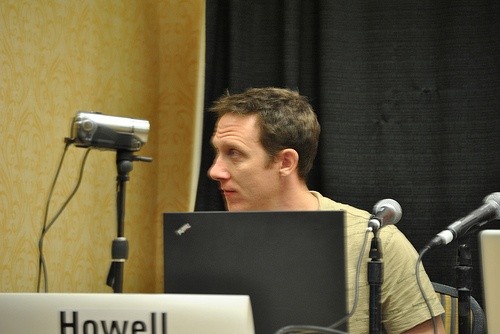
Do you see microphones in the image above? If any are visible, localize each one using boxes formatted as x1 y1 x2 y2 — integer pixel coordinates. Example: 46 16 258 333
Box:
366 199 403 232
426 192 500 246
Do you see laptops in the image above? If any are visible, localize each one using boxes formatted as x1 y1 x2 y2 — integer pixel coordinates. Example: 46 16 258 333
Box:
479 229 500 334
162 211 349 334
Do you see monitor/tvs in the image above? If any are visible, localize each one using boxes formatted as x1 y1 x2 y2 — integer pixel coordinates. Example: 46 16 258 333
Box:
0 293 255 333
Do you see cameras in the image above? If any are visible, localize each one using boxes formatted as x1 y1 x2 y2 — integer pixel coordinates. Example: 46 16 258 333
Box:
74 113 149 152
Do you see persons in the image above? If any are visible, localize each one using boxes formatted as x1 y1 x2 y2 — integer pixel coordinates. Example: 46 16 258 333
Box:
204 87 446 333
